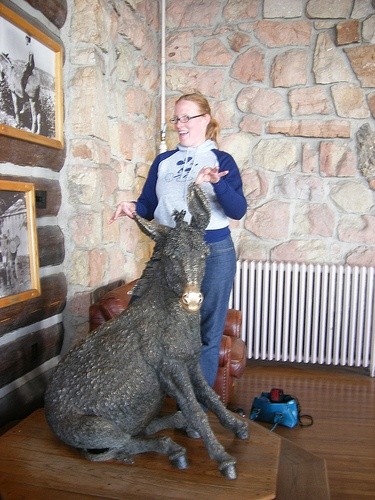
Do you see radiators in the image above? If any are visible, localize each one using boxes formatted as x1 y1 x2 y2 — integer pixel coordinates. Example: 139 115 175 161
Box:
226 258 375 378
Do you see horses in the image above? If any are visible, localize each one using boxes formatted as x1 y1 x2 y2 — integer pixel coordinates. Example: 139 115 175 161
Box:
44 181 249 481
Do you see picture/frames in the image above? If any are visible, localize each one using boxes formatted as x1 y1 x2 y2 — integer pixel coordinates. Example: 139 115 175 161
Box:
0 180 42 309
0 2 68 150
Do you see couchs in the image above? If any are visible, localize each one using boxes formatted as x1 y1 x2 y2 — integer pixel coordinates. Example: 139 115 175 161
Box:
88 278 248 410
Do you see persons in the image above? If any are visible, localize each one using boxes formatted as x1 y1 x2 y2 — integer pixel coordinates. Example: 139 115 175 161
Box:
108 94 247 439
21 36 35 103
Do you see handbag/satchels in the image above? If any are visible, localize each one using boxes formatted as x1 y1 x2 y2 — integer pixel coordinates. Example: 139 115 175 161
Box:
249 389 300 428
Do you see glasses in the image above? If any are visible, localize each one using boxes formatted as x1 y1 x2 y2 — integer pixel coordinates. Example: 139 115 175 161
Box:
169 113 207 124
